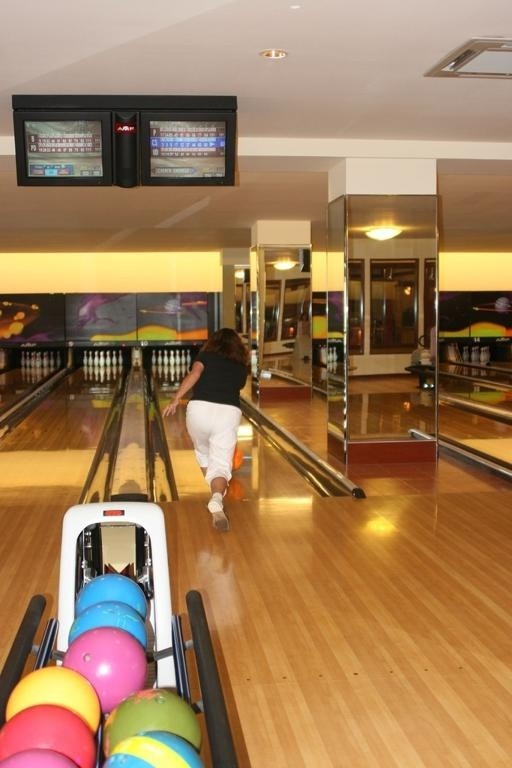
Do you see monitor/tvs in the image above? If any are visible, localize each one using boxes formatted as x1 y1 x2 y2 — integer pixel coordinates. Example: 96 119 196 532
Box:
13 111 113 186
140 111 236 187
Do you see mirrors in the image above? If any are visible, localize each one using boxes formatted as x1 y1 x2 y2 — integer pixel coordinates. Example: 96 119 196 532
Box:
233 265 252 369
345 193 438 444
262 244 312 388
423 257 436 350
369 258 419 355
326 196 346 439
349 258 365 355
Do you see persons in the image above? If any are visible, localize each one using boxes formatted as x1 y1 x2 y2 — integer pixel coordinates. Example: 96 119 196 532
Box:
160 328 250 533
300 312 310 362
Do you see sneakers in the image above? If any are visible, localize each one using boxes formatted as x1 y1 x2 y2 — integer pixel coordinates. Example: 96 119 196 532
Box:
208 498 229 531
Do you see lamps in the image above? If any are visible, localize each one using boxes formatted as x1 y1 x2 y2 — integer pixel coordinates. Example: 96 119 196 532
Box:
235 270 244 279
366 228 402 241
273 261 295 271
